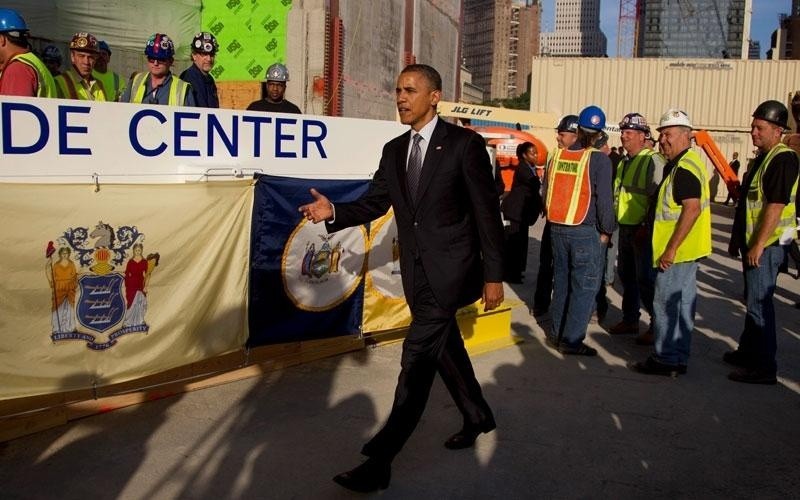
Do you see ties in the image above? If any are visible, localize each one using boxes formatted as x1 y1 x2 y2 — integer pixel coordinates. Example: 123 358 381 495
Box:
406 134 423 205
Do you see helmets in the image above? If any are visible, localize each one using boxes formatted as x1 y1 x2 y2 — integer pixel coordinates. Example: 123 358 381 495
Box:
752 100 792 130
190 31 219 53
69 32 100 52
0 7 30 32
41 46 63 63
98 41 112 54
264 63 289 82
655 108 693 131
619 113 650 133
554 115 578 132
144 33 175 58
577 106 606 131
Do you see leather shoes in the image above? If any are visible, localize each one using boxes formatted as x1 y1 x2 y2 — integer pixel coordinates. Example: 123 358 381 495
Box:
332 459 392 492
444 414 498 450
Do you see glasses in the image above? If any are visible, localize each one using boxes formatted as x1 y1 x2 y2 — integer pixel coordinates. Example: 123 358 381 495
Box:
148 56 171 64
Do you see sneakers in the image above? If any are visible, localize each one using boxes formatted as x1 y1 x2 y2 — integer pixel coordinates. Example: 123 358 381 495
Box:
634 326 657 345
728 367 777 386
722 350 752 363
559 342 596 357
607 313 640 335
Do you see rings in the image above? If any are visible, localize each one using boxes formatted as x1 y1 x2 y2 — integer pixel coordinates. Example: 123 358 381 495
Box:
497 304 500 306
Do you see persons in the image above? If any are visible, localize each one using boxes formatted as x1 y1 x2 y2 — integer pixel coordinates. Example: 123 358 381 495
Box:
0 8 57 98
246 63 301 114
298 64 511 493
41 45 63 76
180 32 219 108
122 243 148 327
91 40 126 101
53 31 107 102
46 247 78 334
119 33 196 107
499 101 799 384
